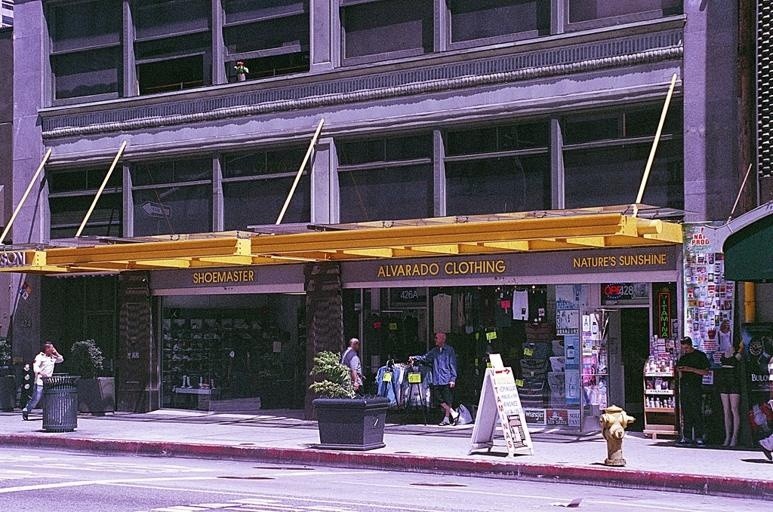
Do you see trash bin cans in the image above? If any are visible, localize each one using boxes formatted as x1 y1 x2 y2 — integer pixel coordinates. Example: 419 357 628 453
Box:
40 375 80 432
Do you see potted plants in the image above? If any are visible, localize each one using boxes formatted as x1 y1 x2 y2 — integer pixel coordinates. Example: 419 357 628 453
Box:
68 338 116 417
306 350 390 451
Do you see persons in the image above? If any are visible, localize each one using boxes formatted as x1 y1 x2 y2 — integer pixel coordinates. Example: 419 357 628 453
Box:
340 337 365 395
674 336 712 447
22 343 65 421
757 338 773 460
715 336 743 446
408 332 461 426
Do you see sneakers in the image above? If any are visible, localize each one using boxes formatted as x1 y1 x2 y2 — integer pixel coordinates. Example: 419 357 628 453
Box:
23 409 29 420
696 440 706 448
675 438 692 447
759 441 772 461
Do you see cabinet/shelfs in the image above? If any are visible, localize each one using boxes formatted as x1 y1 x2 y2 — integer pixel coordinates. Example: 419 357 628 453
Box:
642 358 677 431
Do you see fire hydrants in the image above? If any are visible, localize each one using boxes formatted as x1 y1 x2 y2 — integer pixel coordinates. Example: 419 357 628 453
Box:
598 403 637 467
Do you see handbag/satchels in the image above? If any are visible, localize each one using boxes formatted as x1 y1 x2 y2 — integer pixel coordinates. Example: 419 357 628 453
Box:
456 404 473 425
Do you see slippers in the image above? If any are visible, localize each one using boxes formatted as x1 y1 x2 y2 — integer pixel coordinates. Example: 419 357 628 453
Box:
453 413 460 426
439 421 450 426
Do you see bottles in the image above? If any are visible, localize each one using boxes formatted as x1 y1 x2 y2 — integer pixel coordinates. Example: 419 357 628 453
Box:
568 373 577 397
592 320 599 335
645 358 676 409
567 343 576 359
